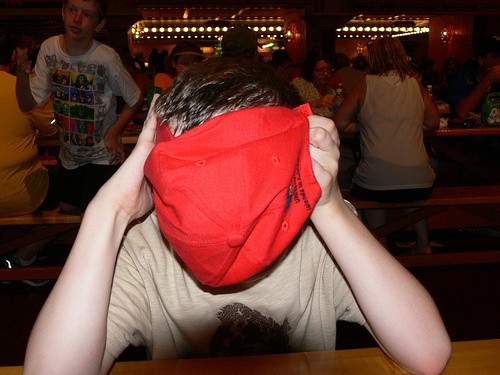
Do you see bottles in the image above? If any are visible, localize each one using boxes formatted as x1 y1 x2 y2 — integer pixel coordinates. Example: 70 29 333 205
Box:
481 83 500 127
332 81 345 115
426 84 435 104
127 51 150 73
136 98 148 125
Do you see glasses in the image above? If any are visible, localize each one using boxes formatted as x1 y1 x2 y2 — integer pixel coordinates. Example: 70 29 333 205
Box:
317 69 331 73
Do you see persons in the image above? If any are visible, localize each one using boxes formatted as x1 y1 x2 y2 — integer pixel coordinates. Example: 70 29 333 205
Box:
406 35 500 184
257 55 272 71
154 43 206 96
222 28 263 65
0 30 59 286
12 0 145 218
271 49 292 68
23 57 452 375
332 37 440 253
290 52 369 119
149 48 169 73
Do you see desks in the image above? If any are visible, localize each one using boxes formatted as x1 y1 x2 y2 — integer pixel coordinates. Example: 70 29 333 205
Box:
38 136 136 148
424 119 500 136
0 338 500 375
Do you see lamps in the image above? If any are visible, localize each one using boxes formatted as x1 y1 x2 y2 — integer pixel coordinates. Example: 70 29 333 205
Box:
439 24 451 45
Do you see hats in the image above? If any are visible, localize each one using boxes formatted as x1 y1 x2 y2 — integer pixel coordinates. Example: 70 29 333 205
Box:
143 103 322 289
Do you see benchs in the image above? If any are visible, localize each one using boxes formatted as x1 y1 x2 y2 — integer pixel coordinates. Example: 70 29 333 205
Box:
0 210 86 280
342 185 500 267
